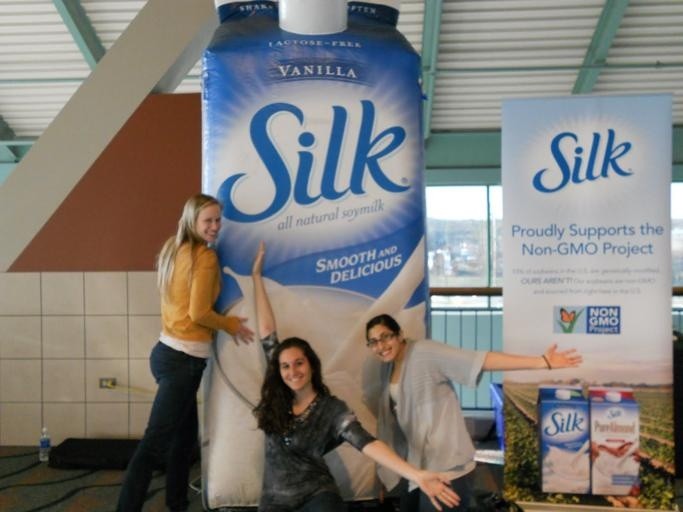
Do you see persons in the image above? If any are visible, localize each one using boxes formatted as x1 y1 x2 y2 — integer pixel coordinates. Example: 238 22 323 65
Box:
113 192 257 511
364 313 583 512
252 239 461 512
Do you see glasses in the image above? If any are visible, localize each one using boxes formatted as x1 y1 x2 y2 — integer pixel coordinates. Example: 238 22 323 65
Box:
366 332 396 347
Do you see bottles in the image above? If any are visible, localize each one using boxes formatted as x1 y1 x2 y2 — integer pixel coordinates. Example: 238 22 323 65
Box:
39 427 50 461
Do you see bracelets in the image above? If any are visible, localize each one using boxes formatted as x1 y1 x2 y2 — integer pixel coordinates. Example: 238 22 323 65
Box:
541 355 553 371
